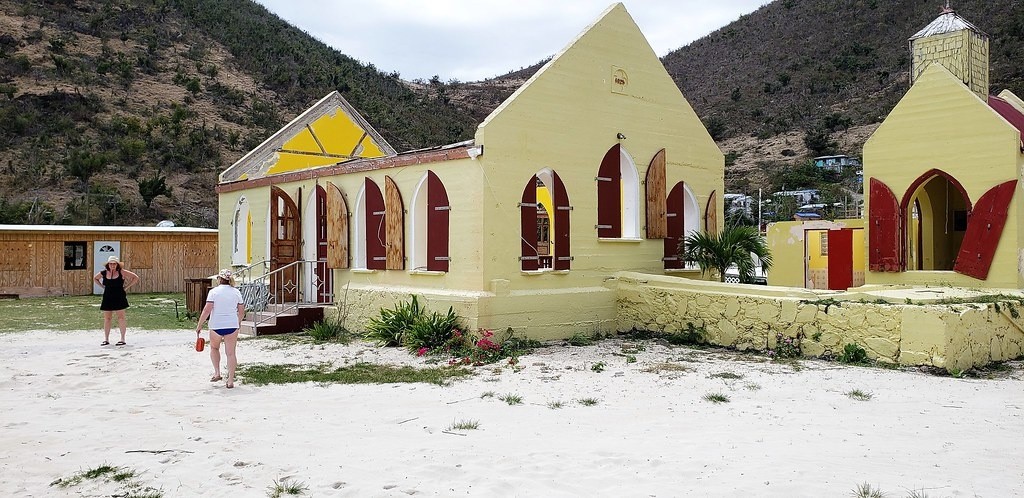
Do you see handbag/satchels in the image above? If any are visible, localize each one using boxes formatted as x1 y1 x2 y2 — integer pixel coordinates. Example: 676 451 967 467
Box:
196 338 205 351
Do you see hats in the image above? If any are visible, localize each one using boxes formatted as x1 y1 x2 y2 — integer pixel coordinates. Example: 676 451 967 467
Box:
219 269 231 281
101 256 124 269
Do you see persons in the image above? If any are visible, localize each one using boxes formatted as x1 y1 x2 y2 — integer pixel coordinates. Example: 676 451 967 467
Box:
195 268 245 389
92 255 140 346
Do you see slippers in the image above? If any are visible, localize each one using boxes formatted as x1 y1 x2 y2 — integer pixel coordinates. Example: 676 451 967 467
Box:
226 380 233 388
210 376 222 382
100 341 109 345
116 342 126 346
